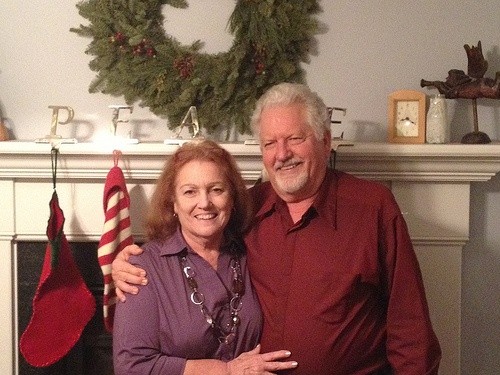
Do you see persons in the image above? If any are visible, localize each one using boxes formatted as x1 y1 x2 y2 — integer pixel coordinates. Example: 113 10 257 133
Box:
112 77 445 375
110 139 299 375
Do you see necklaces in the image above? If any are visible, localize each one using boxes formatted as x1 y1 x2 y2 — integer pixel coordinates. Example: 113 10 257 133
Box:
172 245 247 348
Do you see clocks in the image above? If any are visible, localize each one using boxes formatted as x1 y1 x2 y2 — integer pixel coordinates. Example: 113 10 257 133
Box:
389 89 426 143
